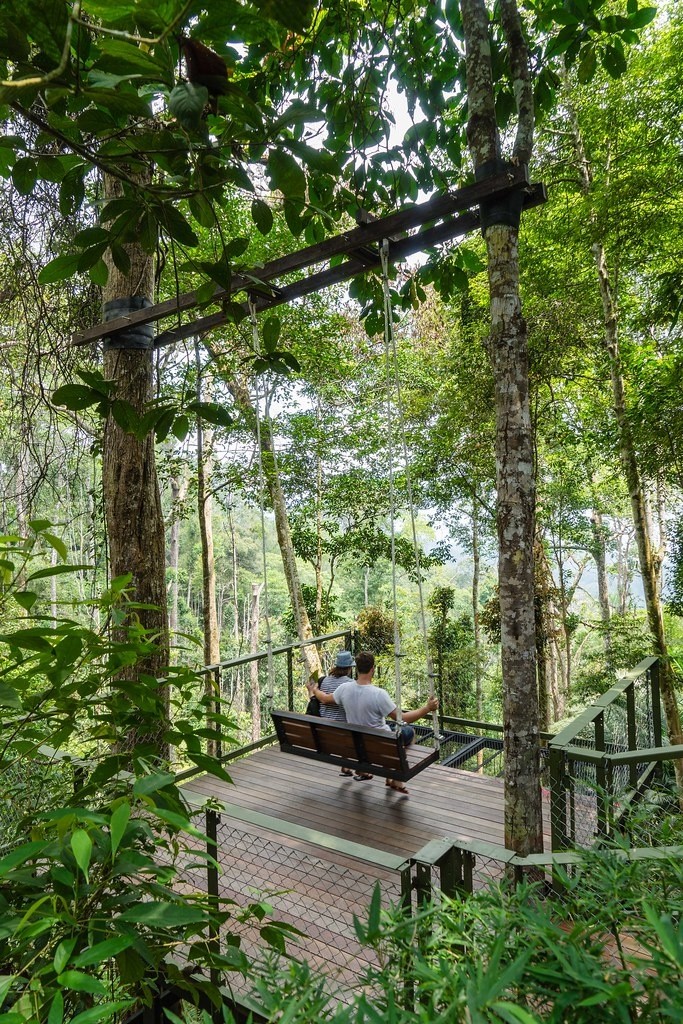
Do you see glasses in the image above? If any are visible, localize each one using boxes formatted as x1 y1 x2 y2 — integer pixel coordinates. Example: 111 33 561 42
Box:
373 665 376 668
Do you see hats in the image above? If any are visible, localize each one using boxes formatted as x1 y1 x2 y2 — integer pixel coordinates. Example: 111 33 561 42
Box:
333 651 357 667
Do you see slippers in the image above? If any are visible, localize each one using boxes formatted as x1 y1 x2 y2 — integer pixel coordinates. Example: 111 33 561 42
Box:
391 786 410 794
385 780 391 786
339 772 353 776
353 773 373 781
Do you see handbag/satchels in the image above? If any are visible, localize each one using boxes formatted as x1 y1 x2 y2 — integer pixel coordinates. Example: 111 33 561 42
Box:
306 676 327 717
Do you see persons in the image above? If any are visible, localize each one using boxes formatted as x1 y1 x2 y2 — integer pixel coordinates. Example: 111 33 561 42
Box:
313 652 439 794
318 651 372 781
306 677 325 717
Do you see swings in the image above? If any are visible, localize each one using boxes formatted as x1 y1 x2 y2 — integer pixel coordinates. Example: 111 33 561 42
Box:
245 237 444 782
